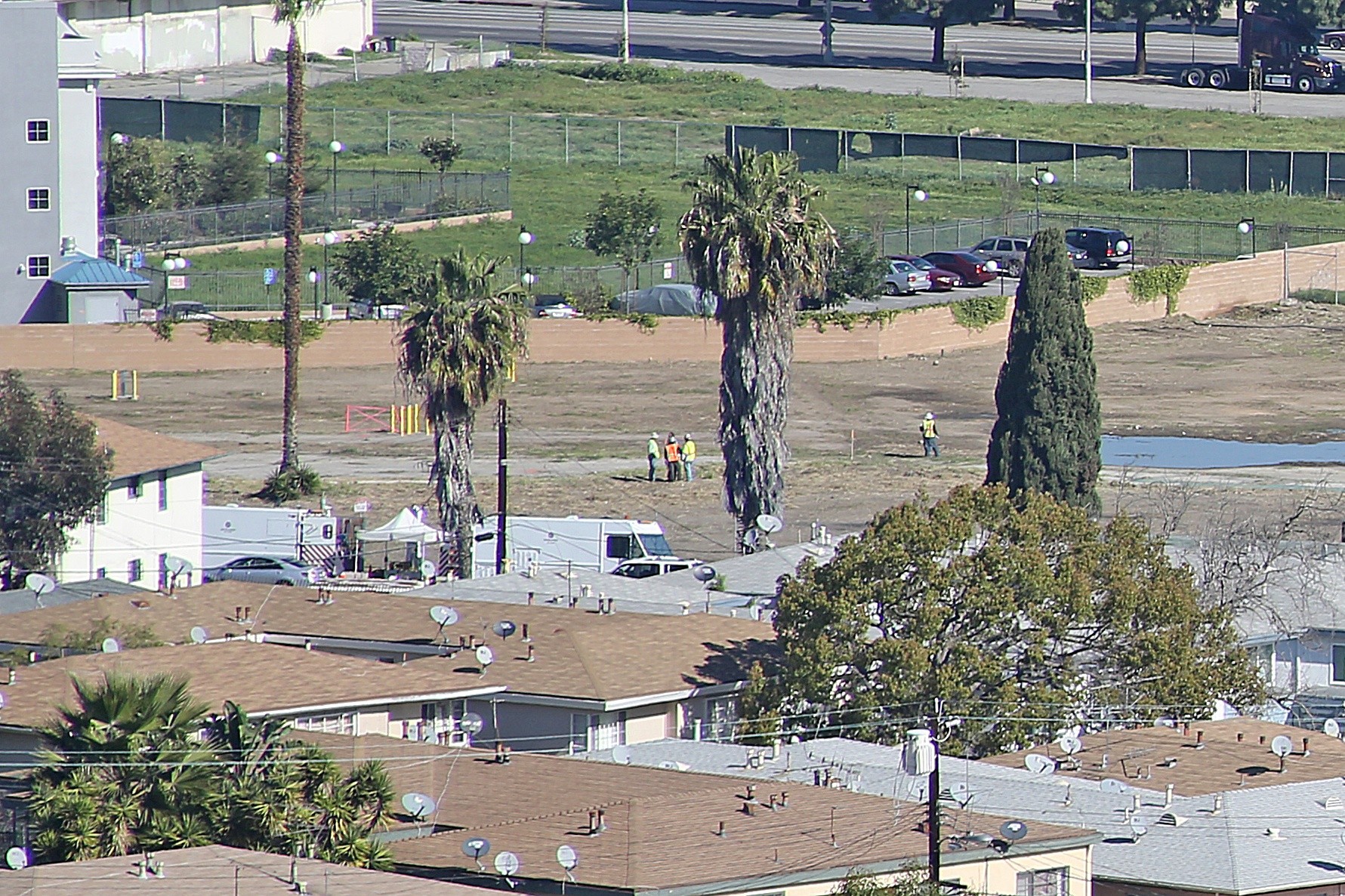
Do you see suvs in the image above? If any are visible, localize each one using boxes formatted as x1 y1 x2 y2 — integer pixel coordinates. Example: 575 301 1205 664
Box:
610 556 704 580
347 298 407 319
528 294 575 318
1065 226 1132 269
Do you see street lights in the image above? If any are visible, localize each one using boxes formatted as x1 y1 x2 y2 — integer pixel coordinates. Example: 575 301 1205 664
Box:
266 152 277 238
104 131 123 217
1030 166 1054 231
1236 217 1255 258
906 183 925 254
161 249 186 316
329 140 342 222
306 226 338 322
517 224 534 295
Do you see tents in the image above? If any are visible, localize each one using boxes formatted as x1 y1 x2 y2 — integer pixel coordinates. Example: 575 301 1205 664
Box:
354 508 457 581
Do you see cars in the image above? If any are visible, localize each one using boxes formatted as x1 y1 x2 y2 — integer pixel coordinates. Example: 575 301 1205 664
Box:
871 236 1088 296
157 302 207 322
203 554 327 587
1319 31 1345 49
610 284 718 317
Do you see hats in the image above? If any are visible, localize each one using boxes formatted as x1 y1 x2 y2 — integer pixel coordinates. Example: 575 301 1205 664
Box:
925 412 934 420
650 432 659 438
684 434 692 440
669 436 677 442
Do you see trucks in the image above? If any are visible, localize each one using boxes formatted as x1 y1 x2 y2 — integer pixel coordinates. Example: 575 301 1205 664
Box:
472 515 671 580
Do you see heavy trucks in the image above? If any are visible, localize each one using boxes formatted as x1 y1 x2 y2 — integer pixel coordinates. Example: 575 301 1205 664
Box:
1180 13 1342 92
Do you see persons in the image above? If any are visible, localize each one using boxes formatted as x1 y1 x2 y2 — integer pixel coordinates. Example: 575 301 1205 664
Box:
920 412 940 458
664 432 695 482
647 432 660 482
